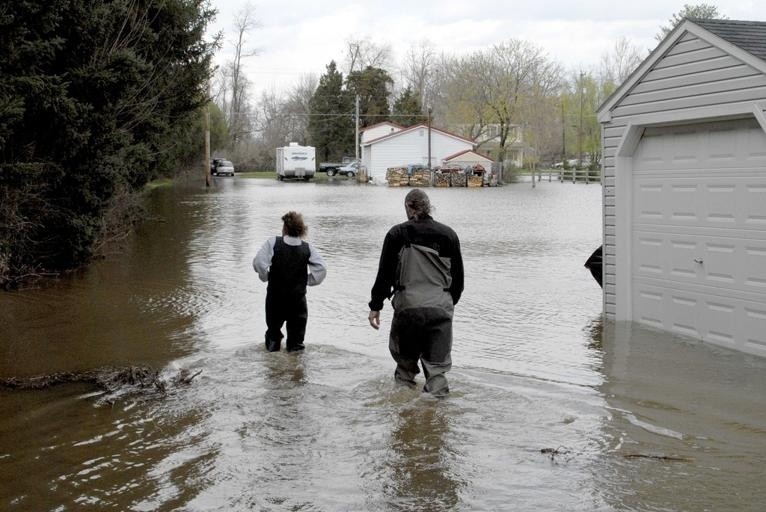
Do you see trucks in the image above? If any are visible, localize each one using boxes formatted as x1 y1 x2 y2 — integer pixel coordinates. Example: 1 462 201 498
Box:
274 141 316 181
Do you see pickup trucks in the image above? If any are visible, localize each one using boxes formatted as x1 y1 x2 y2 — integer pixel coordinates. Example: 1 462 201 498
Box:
319 156 354 176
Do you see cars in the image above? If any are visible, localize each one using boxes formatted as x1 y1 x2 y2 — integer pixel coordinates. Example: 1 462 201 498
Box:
338 161 358 177
211 157 235 177
554 159 578 169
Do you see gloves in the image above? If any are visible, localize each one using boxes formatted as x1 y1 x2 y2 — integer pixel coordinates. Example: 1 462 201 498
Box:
368 311 380 329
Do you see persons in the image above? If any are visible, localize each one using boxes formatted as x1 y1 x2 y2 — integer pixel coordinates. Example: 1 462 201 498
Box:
251 210 328 354
366 188 465 399
